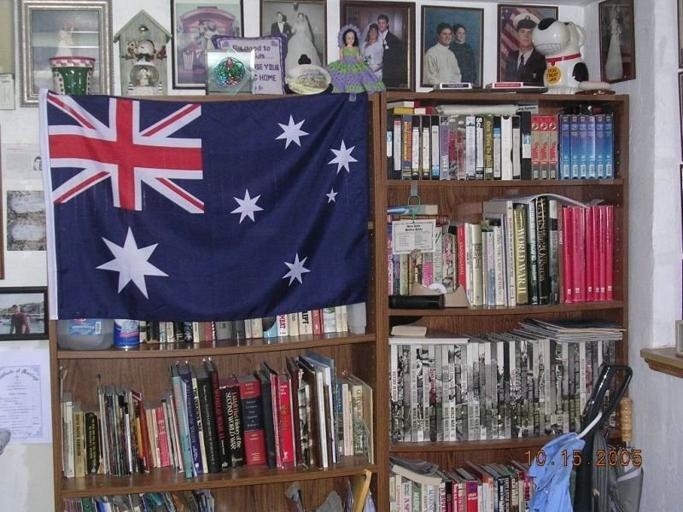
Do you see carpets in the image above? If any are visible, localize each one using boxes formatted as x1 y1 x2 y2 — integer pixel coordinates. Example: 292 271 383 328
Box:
517 55 525 81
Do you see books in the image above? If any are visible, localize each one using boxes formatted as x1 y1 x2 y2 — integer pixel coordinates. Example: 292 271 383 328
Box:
387 194 619 309
387 101 618 181
57 305 367 349
61 353 375 510
387 318 625 510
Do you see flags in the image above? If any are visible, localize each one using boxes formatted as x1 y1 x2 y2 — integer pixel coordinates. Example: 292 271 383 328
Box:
38 89 370 321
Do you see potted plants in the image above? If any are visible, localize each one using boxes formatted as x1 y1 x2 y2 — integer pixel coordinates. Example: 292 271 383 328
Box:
0 286 48 340
171 0 244 89
260 1 327 69
421 5 484 88
340 0 416 92
211 35 286 96
598 1 636 83
13 1 113 108
204 49 255 94
497 3 558 86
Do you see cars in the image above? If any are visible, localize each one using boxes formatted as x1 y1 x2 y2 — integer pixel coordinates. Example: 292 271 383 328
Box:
48 56 96 96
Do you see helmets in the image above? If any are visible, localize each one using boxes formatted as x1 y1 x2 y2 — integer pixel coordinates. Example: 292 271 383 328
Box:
286 64 331 95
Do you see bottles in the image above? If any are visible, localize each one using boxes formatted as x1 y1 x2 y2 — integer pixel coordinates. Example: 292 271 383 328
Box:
513 13 540 32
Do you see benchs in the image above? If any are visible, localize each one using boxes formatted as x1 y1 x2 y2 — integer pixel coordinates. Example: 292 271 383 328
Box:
38 89 370 321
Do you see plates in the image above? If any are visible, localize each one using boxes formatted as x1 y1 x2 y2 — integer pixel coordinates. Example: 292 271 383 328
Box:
379 92 629 511
41 93 385 512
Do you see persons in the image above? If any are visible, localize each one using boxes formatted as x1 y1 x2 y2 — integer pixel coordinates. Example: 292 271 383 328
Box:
448 24 477 84
10 304 30 334
604 5 624 82
504 12 546 87
423 23 462 86
270 12 292 81
360 14 403 88
285 13 321 85
